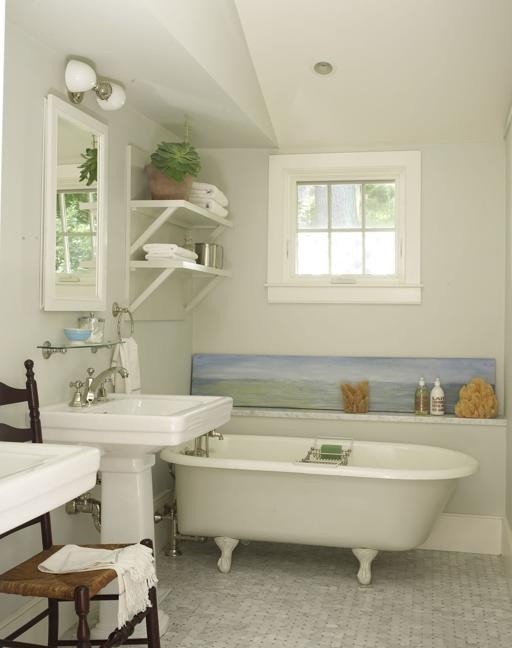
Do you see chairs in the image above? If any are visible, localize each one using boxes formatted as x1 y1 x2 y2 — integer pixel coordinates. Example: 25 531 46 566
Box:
0 359 161 647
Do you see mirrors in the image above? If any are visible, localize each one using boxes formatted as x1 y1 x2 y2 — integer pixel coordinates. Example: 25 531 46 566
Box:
40 94 109 314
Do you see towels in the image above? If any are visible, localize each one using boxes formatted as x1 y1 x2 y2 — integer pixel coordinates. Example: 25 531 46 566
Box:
36 544 159 631
143 244 199 264
189 182 229 217
111 338 143 395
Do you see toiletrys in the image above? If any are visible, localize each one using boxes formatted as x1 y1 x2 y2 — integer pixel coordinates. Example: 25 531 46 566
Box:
430 375 447 416
413 375 431 415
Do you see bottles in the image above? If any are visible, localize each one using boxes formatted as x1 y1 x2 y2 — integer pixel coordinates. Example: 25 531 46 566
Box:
88 312 97 343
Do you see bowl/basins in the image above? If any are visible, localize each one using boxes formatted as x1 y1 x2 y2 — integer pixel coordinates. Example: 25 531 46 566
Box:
194 243 224 269
64 328 91 346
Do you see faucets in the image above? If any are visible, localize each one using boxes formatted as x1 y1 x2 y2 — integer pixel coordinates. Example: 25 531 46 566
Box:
194 429 223 455
80 364 130 406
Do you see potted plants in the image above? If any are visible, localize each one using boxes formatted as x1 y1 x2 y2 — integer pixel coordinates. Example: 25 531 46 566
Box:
146 140 204 200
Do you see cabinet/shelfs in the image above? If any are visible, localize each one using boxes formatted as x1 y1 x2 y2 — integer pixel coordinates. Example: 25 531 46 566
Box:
125 145 232 322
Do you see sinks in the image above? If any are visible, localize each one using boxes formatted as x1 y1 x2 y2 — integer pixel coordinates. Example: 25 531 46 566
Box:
0 440 101 537
25 391 234 455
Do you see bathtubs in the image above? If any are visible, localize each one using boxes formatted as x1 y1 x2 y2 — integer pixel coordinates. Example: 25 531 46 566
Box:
159 434 479 551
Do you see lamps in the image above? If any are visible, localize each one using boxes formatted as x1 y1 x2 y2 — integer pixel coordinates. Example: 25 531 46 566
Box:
64 58 126 112
